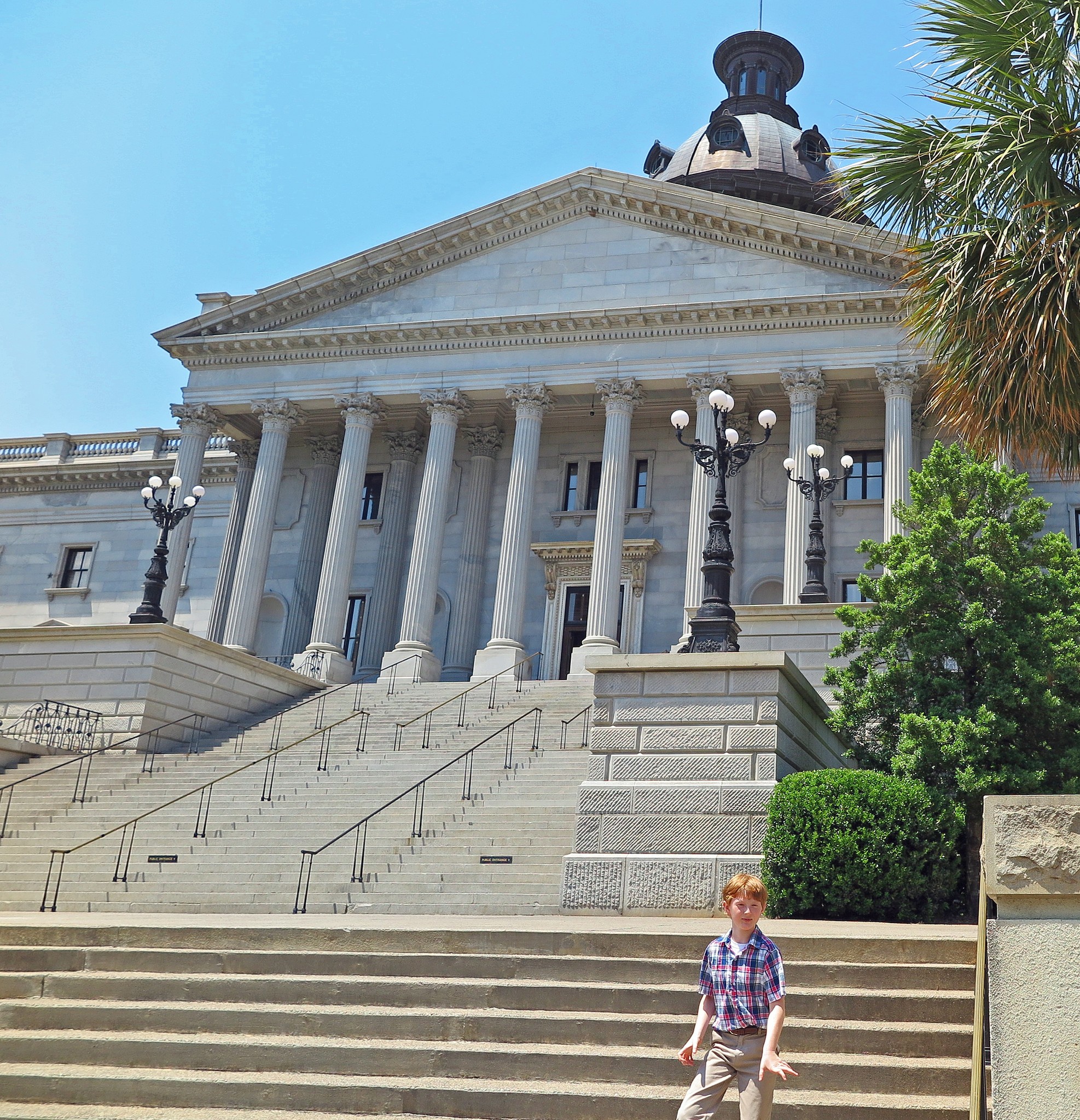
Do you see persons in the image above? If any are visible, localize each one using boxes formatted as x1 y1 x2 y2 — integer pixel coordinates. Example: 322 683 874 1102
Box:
674 873 799 1120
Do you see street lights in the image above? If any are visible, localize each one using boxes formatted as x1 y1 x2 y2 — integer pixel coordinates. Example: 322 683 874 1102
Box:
127 474 203 625
669 388 778 652
782 443 855 602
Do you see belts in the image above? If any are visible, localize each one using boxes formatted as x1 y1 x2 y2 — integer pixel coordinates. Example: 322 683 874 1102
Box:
729 1027 759 1036
